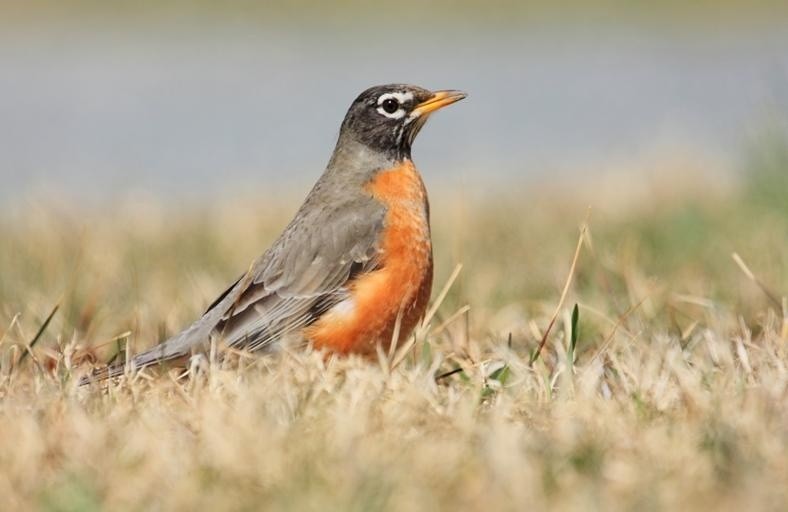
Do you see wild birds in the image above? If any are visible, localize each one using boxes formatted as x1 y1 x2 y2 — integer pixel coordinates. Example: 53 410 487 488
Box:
77 82 470 389
